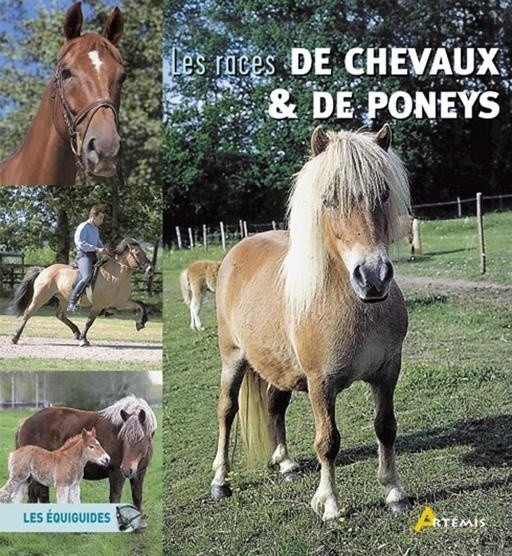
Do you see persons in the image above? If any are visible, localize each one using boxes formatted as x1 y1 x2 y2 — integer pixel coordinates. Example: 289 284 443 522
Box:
66 203 112 313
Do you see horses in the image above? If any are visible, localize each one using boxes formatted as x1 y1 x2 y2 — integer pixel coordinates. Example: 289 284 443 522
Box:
12 392 157 513
207 123 416 525
0 1 129 185
0 427 111 505
179 258 221 334
9 236 156 349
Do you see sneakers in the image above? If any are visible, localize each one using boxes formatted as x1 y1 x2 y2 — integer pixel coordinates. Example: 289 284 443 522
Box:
66 304 78 312
